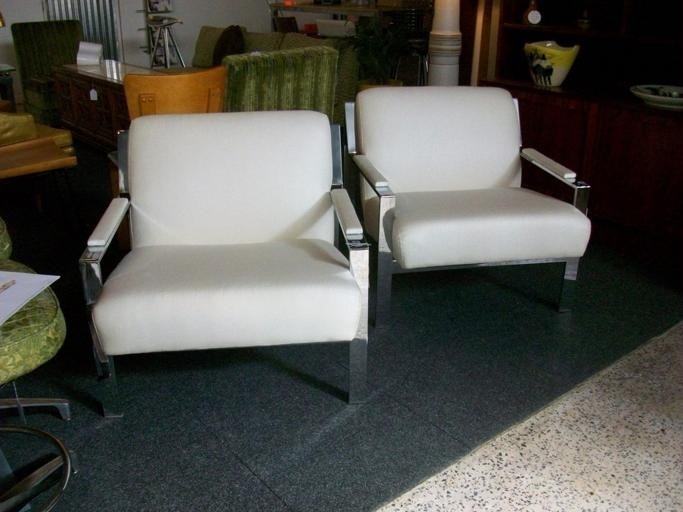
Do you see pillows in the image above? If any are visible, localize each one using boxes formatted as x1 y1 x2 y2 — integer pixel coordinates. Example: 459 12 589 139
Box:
211 25 245 67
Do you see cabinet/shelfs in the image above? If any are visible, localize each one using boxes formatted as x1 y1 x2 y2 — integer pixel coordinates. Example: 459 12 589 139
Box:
494 0 682 96
477 67 682 294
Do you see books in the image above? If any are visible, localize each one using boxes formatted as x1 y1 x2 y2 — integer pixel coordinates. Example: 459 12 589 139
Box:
0 271 62 331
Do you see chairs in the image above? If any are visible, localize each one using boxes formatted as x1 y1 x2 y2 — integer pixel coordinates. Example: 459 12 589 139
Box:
0 219 71 427
10 19 84 129
345 82 591 330
79 110 371 406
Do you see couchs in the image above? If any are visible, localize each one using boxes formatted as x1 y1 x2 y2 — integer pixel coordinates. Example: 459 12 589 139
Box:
153 25 359 147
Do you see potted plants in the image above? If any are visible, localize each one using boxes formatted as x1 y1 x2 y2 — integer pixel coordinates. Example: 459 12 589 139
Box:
349 7 429 92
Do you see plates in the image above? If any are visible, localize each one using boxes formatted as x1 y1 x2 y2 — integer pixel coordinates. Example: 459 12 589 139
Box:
631 85 683 104
